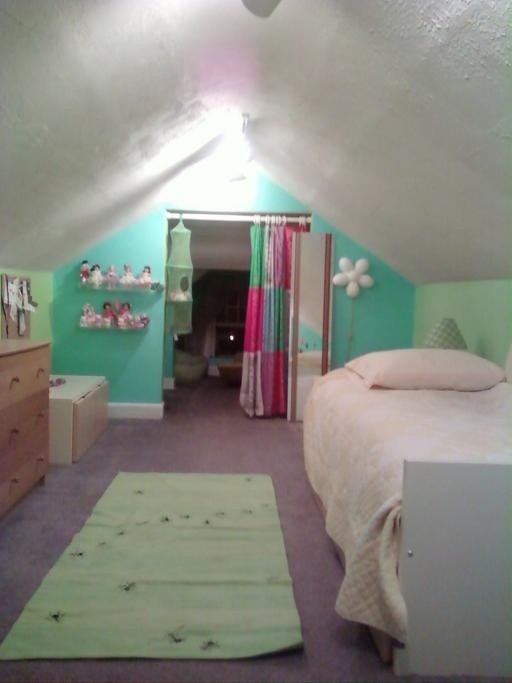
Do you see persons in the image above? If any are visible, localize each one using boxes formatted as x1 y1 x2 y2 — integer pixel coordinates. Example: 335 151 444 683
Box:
78 258 152 330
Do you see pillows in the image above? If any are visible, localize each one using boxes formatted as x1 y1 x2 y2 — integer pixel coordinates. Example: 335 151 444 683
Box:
343 348 507 391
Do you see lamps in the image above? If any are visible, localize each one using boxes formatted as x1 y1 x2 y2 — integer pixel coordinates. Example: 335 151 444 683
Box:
424 317 469 351
332 257 375 365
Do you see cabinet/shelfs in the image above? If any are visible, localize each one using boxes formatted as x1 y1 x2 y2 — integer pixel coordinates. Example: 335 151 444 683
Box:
49 375 109 465
0 338 51 521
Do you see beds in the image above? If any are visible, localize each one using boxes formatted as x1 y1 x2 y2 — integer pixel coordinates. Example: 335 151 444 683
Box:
303 367 512 683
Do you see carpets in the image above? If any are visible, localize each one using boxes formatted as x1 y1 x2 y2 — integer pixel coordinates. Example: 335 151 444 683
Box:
0 471 305 661
